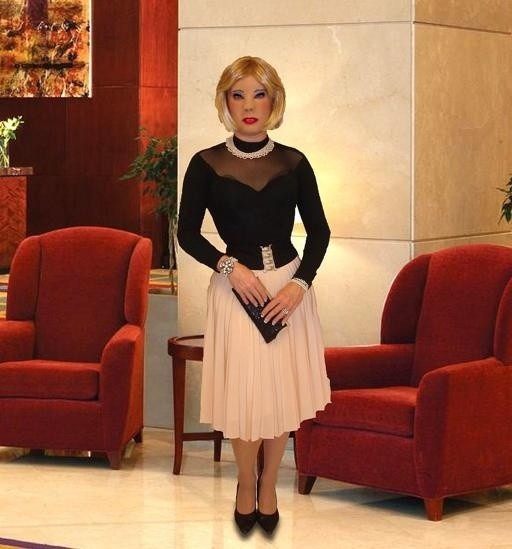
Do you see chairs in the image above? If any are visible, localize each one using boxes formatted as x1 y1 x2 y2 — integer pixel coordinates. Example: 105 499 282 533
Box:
1 226 150 471
293 242 510 520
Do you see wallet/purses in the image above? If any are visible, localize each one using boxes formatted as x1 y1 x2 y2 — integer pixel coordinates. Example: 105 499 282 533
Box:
231 278 287 343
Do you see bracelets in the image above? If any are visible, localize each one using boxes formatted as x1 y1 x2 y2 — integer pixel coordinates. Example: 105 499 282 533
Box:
292 278 310 294
216 256 239 278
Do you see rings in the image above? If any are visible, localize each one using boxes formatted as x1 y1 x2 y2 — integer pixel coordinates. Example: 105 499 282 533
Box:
282 309 289 315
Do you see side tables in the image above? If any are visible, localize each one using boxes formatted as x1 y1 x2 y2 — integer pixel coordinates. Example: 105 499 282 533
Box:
169 334 228 477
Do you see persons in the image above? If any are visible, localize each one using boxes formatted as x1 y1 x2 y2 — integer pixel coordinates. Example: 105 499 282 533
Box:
177 54 331 536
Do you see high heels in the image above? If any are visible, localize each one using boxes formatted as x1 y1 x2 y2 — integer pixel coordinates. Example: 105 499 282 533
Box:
234 470 279 535
